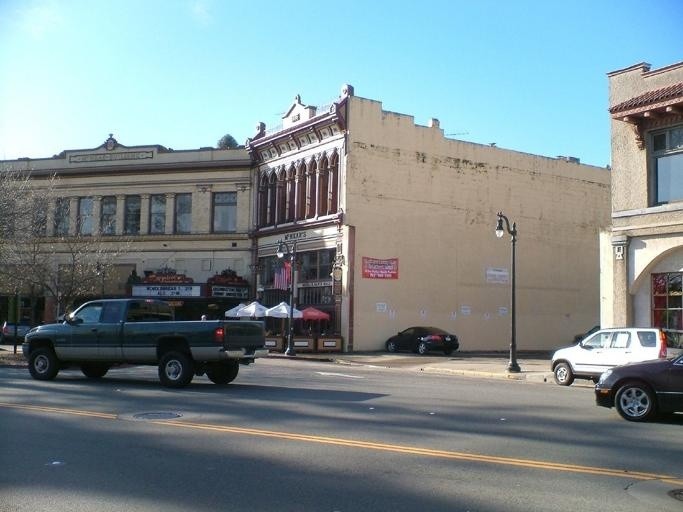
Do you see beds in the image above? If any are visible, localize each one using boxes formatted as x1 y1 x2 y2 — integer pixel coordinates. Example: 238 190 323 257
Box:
225 301 330 334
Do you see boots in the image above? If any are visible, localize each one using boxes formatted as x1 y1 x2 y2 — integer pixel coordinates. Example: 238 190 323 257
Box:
549 328 682 387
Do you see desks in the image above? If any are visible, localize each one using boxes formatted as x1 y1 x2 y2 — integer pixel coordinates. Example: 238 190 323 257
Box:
274 239 300 359
494 211 522 374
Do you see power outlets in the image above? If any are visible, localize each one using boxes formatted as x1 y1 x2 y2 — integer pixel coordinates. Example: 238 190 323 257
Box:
22 296 270 391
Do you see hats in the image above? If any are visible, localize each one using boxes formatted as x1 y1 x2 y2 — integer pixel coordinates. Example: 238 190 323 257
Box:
384 326 460 356
2 322 30 339
595 354 682 421
